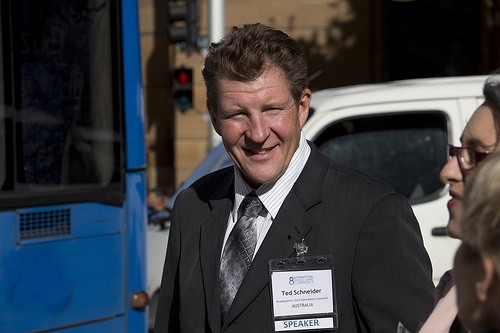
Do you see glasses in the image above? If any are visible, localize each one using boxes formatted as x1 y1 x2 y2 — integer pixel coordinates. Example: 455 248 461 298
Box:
445 144 499 171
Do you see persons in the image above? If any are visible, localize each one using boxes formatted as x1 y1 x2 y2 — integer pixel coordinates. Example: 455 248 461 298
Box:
152 24 438 333
418 73 500 333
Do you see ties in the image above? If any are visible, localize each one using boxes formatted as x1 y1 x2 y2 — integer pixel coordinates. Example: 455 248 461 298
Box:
218 189 263 325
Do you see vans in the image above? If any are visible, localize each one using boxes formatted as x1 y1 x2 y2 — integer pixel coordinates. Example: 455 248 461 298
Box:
147 76 500 331
